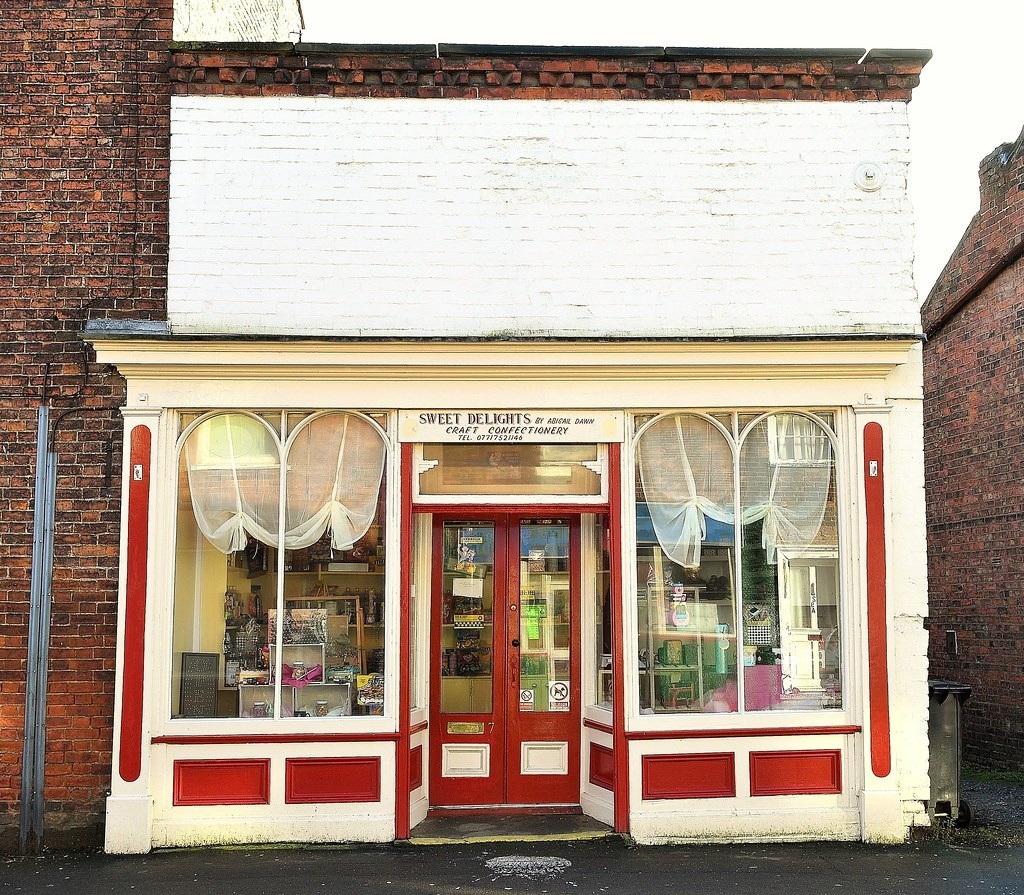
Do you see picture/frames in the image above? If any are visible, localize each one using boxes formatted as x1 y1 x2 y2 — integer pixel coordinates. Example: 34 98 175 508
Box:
180 652 220 717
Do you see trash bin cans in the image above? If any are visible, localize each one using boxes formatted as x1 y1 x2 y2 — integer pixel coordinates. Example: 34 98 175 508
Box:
928 680 975 827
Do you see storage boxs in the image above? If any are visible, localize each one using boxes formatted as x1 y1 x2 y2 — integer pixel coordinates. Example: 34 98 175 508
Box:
357 675 384 706
269 643 326 684
328 563 370 572
238 684 295 718
295 684 351 716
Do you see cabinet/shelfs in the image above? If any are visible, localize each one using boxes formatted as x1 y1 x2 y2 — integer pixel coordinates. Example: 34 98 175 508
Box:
172 511 736 717
